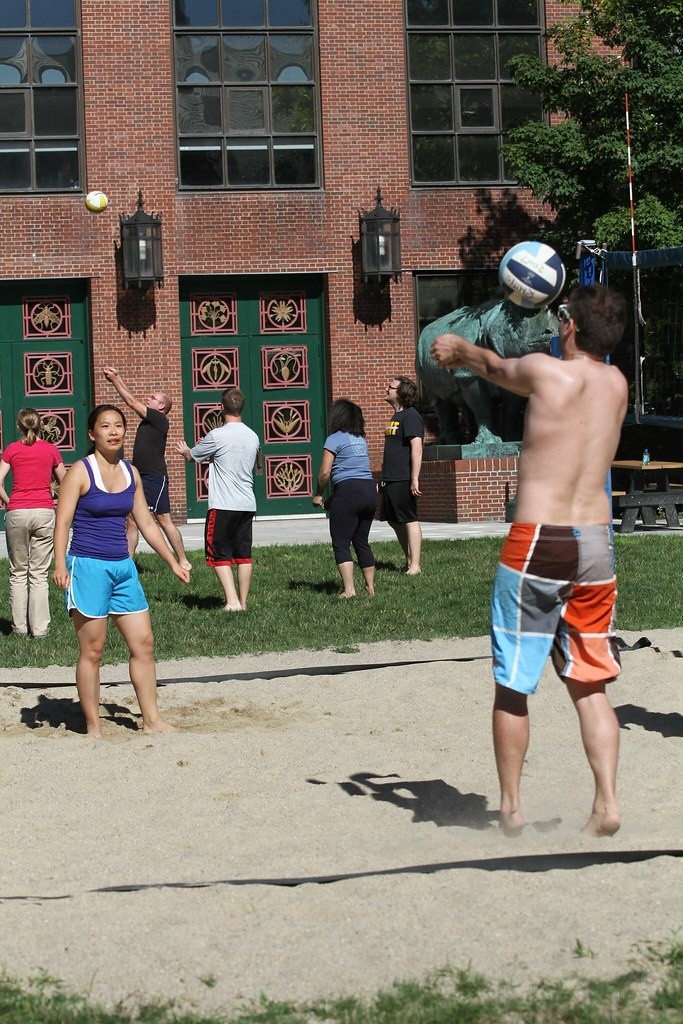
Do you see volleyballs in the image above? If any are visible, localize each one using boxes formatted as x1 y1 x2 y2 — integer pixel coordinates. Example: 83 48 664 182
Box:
84 191 108 213
496 240 567 310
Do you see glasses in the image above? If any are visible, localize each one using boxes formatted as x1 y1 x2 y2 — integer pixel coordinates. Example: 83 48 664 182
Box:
388 385 397 390
557 304 579 331
150 395 164 404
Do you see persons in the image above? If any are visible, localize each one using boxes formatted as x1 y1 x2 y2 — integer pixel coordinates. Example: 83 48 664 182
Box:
430 284 629 837
311 400 377 599
50 404 190 737
175 388 259 611
380 375 425 574
103 367 192 571
0 408 66 636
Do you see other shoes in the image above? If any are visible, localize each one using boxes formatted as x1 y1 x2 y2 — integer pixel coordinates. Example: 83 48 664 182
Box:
35 635 45 639
13 633 27 637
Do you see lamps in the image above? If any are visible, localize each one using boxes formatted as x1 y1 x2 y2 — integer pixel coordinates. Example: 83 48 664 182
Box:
358 184 403 294
114 190 164 300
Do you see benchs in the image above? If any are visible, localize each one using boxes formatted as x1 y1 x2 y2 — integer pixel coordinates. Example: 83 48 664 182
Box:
611 483 683 534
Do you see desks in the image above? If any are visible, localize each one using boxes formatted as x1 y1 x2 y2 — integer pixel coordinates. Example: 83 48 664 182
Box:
611 460 683 524
